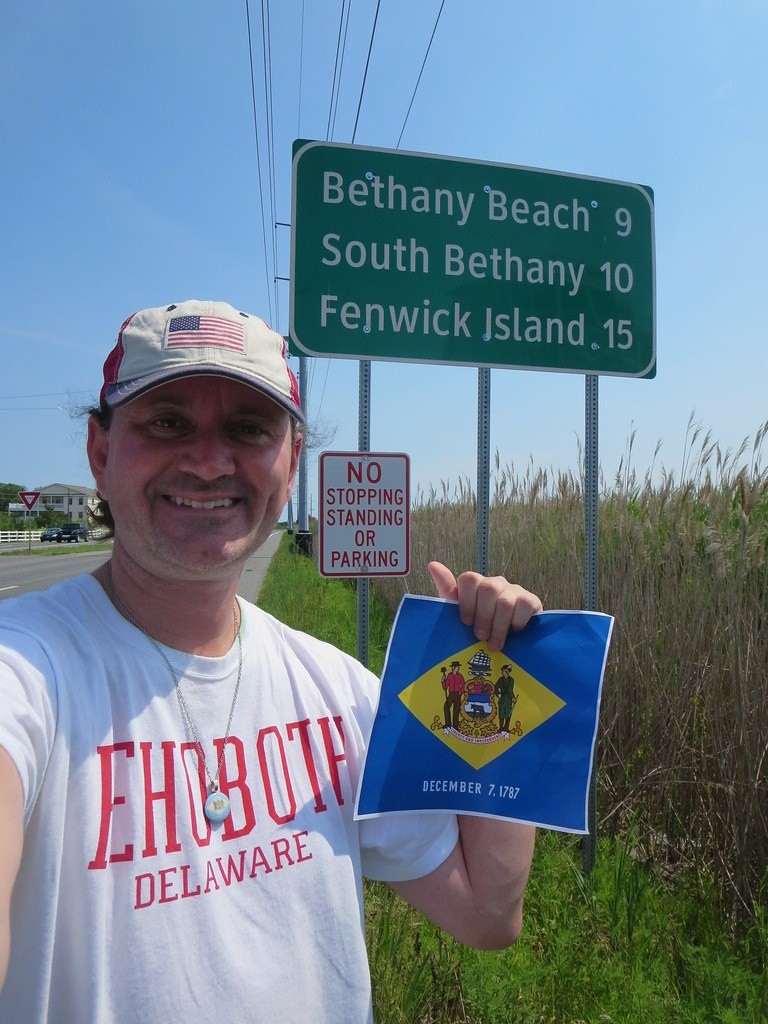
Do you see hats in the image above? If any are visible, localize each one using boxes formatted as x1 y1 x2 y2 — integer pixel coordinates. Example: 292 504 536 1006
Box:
100 300 305 427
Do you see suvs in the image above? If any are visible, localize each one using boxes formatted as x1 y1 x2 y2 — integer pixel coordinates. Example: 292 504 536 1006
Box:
57 522 89 542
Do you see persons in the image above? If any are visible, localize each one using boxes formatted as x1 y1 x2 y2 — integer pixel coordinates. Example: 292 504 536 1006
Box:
0 299 544 1024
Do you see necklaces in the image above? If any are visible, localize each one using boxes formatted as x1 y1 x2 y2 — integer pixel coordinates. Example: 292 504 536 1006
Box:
106 559 243 823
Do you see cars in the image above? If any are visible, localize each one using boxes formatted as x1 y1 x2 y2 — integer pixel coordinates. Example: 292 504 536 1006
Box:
40 528 61 542
